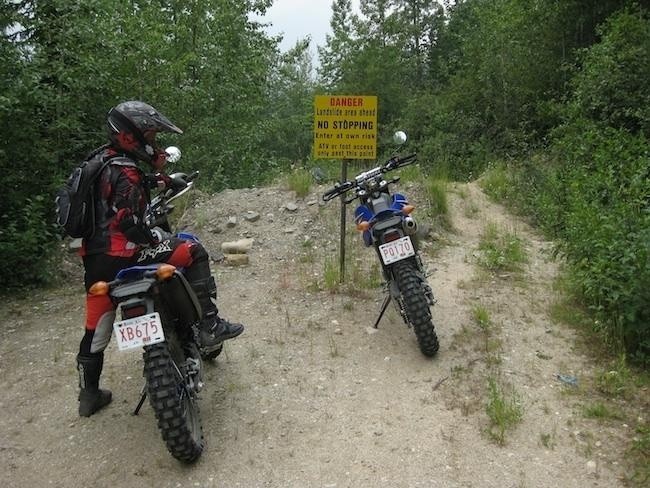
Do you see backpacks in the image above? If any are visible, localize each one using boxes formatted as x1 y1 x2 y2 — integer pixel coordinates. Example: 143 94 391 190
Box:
52 142 137 239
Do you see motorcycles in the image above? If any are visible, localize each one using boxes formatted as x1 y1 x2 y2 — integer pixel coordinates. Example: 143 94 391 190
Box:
68 144 207 467
321 130 441 359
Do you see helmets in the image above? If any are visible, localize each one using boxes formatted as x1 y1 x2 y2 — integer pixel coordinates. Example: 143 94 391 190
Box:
108 100 183 170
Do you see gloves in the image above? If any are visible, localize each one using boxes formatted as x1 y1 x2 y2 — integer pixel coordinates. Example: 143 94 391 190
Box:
151 225 174 244
168 172 188 189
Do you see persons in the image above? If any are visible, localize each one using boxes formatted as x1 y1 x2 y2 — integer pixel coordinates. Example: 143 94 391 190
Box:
72 100 244 418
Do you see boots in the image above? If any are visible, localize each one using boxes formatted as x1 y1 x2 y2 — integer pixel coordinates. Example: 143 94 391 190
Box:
76 352 113 417
192 276 244 346
